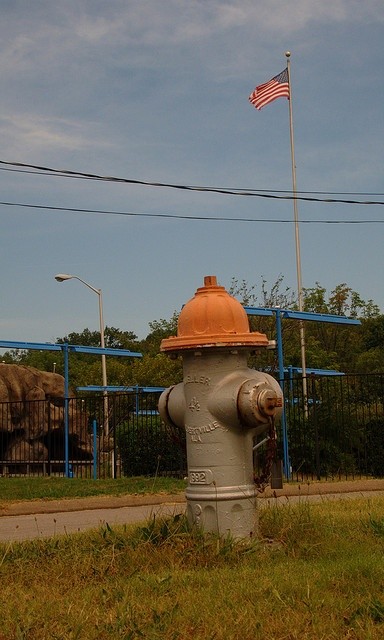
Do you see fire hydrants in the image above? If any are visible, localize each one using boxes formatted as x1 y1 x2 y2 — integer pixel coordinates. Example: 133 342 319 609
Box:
159 276 284 546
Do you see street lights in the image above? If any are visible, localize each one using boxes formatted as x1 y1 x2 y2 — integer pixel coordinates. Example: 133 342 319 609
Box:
55 274 110 480
